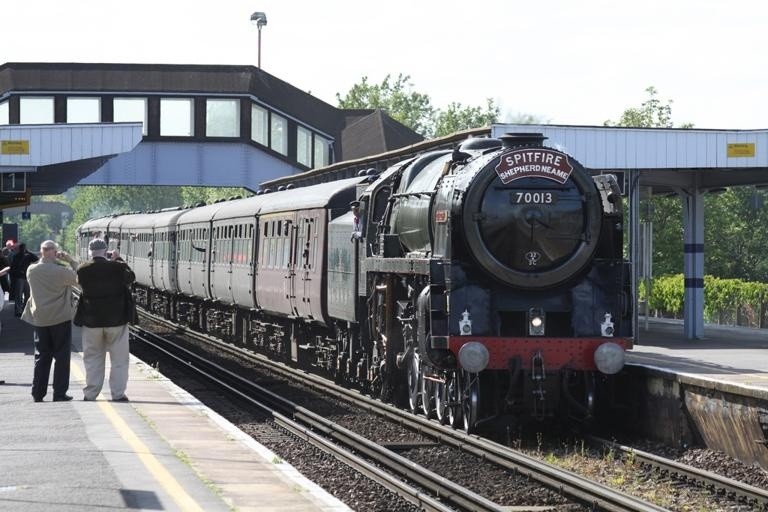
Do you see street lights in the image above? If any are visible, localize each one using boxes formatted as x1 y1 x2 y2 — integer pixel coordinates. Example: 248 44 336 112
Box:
249 7 271 69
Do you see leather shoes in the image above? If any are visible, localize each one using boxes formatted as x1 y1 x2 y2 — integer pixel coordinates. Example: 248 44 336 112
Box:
53 395 73 401
113 395 128 401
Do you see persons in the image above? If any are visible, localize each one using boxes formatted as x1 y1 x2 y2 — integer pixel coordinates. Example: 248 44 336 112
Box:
76 239 139 402
0 239 39 317
21 241 80 402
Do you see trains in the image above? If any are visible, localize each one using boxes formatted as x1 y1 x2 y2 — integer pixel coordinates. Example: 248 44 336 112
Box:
73 125 635 448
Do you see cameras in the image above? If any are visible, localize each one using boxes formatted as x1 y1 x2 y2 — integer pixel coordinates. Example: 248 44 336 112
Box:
56 253 65 258
107 252 112 258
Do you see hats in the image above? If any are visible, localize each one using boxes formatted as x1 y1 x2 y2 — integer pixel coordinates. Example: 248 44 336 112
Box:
89 239 106 250
41 240 58 250
